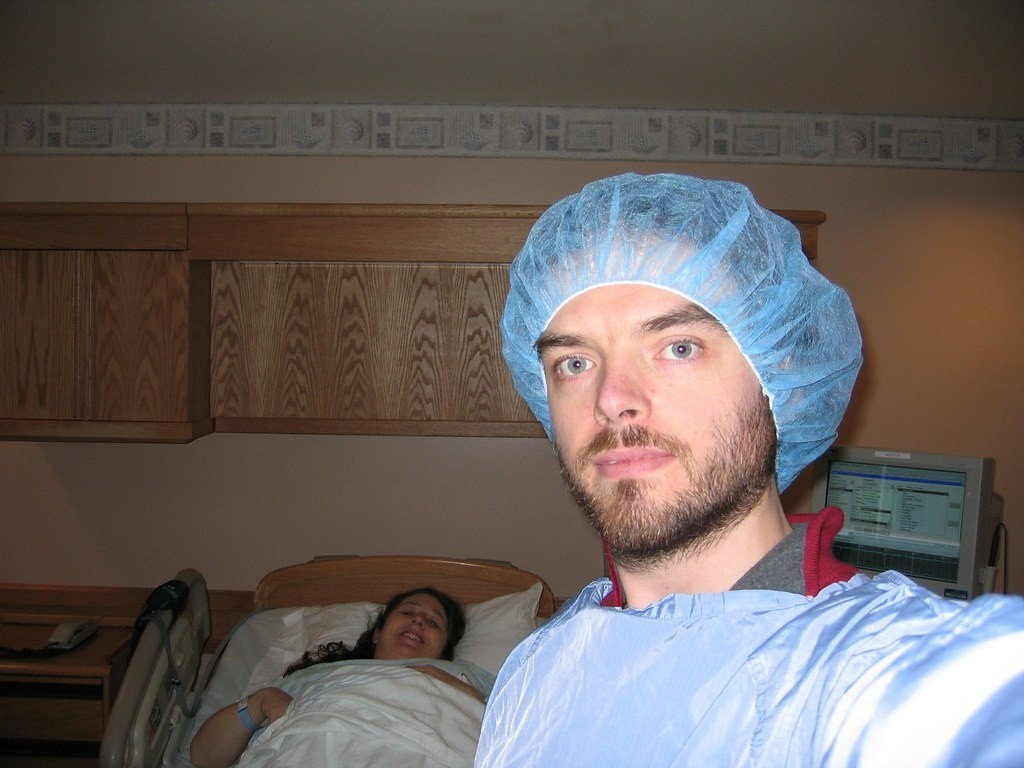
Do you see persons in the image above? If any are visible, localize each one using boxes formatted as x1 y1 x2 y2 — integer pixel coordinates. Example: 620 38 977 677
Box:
474 174 1024 768
190 585 496 768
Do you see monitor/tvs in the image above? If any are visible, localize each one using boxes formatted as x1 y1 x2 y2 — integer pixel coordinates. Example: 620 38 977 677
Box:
811 445 1004 602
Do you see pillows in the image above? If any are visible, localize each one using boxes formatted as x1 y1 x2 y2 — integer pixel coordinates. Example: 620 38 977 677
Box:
302 581 543 677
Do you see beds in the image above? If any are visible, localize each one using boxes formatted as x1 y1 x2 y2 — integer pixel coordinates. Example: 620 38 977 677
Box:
97 554 556 768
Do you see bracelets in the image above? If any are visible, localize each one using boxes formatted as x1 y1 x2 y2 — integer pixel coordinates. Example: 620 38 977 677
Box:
237 697 268 731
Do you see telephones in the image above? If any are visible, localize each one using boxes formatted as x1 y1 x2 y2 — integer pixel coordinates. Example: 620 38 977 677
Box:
47 619 99 650
